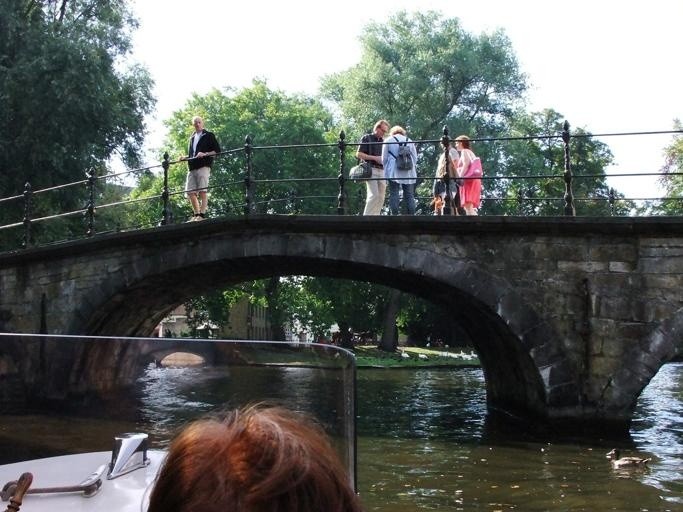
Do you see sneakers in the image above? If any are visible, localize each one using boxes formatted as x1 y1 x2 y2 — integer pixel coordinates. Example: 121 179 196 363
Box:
185 216 206 223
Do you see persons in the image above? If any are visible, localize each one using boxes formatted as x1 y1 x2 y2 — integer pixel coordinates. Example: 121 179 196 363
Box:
354 117 390 216
380 125 417 214
453 134 482 216
176 114 222 222
136 396 367 512
431 133 458 215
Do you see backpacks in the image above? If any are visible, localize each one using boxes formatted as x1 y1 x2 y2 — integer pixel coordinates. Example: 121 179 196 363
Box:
388 136 414 171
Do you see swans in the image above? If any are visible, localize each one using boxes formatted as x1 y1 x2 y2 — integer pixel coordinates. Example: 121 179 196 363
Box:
419 351 429 360
401 346 410 359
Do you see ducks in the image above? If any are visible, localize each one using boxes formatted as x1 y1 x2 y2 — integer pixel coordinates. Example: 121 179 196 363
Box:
606 448 652 471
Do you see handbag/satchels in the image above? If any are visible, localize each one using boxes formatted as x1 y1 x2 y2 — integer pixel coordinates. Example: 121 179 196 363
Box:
456 155 483 178
349 161 372 183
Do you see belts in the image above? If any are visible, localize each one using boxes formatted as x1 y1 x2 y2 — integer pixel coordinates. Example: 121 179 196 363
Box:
371 164 383 169
